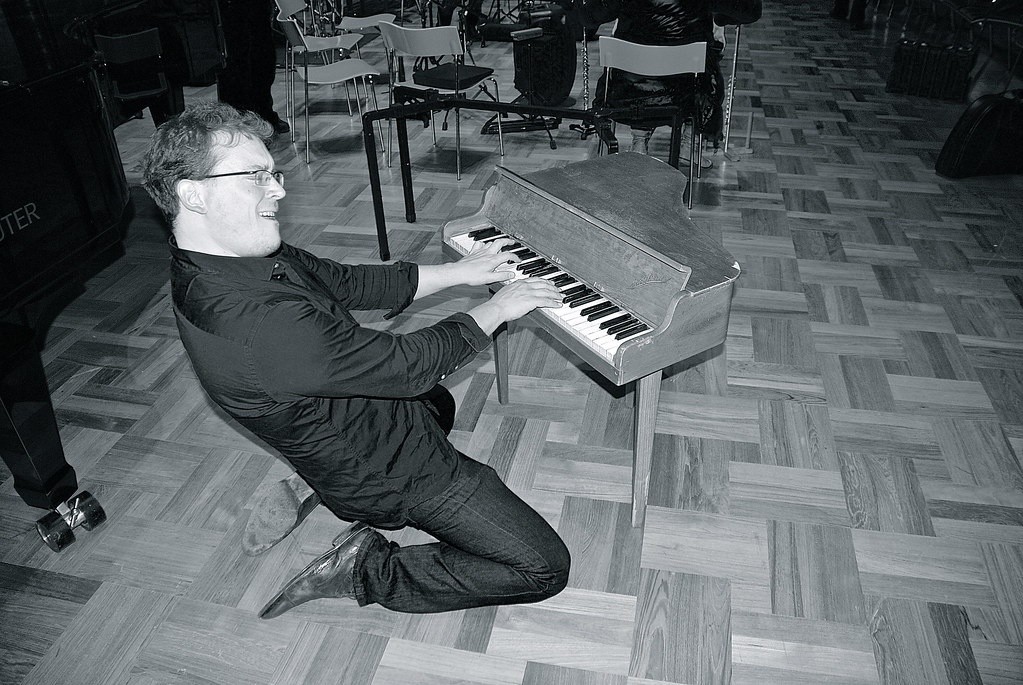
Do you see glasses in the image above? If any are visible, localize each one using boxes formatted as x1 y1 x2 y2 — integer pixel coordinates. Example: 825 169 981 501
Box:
207 169 285 188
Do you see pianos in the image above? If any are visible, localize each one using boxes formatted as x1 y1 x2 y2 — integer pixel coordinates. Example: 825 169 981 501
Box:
1 0 291 553
442 149 741 530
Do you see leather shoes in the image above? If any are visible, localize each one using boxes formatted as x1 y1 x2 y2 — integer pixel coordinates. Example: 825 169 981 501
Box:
257 519 372 620
243 470 322 553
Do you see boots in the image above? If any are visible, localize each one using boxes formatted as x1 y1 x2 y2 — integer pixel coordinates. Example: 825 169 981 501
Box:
679 121 714 170
629 128 652 155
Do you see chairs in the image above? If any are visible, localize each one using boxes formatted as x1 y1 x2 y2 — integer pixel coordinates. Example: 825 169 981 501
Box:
275 0 707 208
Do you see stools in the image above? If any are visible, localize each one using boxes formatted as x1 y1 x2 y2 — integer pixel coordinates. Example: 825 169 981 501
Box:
481 24 527 48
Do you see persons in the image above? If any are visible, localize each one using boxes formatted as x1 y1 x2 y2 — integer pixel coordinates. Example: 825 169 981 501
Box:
436 0 484 41
215 0 290 134
135 104 572 619
565 0 762 169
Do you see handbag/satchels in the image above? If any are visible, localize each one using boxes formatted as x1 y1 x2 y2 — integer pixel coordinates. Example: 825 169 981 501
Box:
936 88 1023 179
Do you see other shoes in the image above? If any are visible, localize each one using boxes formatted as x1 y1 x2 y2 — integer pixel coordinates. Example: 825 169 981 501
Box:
830 9 846 19
277 117 290 133
850 21 872 31
